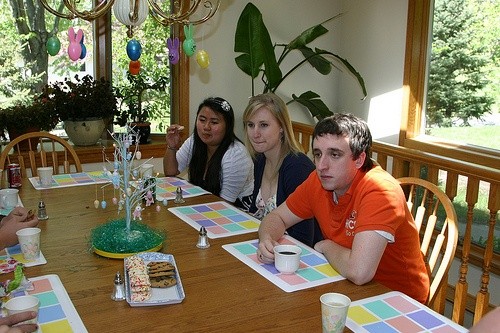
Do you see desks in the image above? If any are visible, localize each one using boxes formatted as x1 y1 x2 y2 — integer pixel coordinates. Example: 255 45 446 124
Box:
0 172 394 333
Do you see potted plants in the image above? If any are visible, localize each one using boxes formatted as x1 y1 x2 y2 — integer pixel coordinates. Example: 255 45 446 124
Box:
111 71 169 144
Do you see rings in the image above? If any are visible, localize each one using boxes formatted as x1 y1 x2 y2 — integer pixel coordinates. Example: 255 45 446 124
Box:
260 255 263 259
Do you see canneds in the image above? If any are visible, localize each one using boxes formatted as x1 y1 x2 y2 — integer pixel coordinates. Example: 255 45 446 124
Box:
7 163 22 189
141 176 157 203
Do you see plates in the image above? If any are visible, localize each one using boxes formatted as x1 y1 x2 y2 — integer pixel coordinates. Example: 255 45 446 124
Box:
123 250 186 307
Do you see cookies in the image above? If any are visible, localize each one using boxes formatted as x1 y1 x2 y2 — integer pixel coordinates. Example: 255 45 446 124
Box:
126 256 152 303
147 261 177 288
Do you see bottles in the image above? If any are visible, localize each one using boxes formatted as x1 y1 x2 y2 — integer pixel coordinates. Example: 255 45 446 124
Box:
111 271 125 302
196 226 210 249
37 200 48 221
174 187 185 203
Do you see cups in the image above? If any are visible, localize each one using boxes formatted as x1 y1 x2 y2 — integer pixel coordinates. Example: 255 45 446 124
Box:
36 167 53 187
272 244 302 275
15 227 42 261
4 295 40 333
320 292 351 333
0 188 19 209
141 164 153 180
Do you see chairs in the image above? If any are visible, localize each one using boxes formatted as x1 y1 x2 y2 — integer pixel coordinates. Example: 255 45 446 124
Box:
396 177 459 308
0 132 83 179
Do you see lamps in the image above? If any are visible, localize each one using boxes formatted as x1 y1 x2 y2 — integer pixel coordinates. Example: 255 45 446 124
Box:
40 0 221 39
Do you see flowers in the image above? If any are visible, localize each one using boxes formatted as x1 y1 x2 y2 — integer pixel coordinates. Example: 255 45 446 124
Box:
0 74 121 141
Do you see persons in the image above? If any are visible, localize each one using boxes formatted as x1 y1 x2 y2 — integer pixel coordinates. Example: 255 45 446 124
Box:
0 207 39 251
256 113 429 304
242 93 316 249
163 97 254 212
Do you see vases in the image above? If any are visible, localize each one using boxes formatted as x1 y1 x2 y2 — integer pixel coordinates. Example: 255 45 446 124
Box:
9 125 41 151
61 116 107 146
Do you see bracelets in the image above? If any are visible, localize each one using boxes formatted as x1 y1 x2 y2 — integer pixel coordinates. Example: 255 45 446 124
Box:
168 145 176 151
